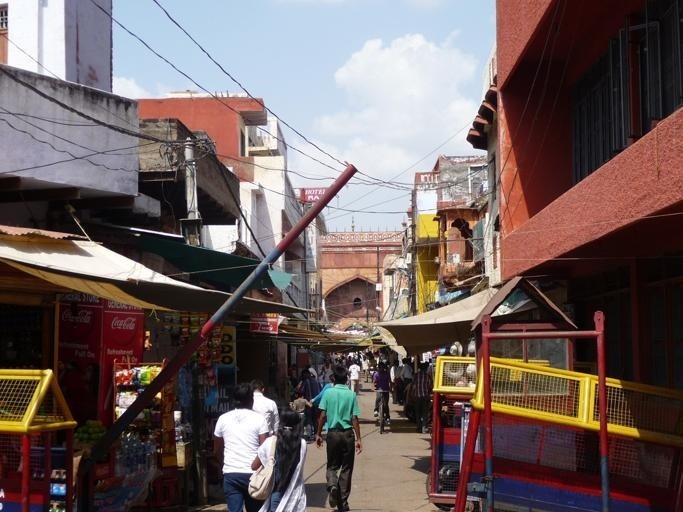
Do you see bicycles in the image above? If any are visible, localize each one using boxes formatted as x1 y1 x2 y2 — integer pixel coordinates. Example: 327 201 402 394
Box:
373 389 391 434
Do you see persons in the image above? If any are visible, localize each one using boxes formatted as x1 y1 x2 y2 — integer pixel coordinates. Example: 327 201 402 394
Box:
250 378 280 436
289 351 433 435
252 409 307 512
214 383 269 512
315 366 362 512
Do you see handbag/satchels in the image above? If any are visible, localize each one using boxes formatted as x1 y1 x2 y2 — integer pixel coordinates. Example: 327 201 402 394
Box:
248 463 277 500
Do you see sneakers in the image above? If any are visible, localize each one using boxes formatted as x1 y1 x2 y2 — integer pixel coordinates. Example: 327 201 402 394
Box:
338 506 349 512
329 486 338 507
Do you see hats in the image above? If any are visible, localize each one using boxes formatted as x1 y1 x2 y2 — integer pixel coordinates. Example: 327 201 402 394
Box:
467 342 476 356
444 363 463 378
449 341 463 357
467 364 476 379
